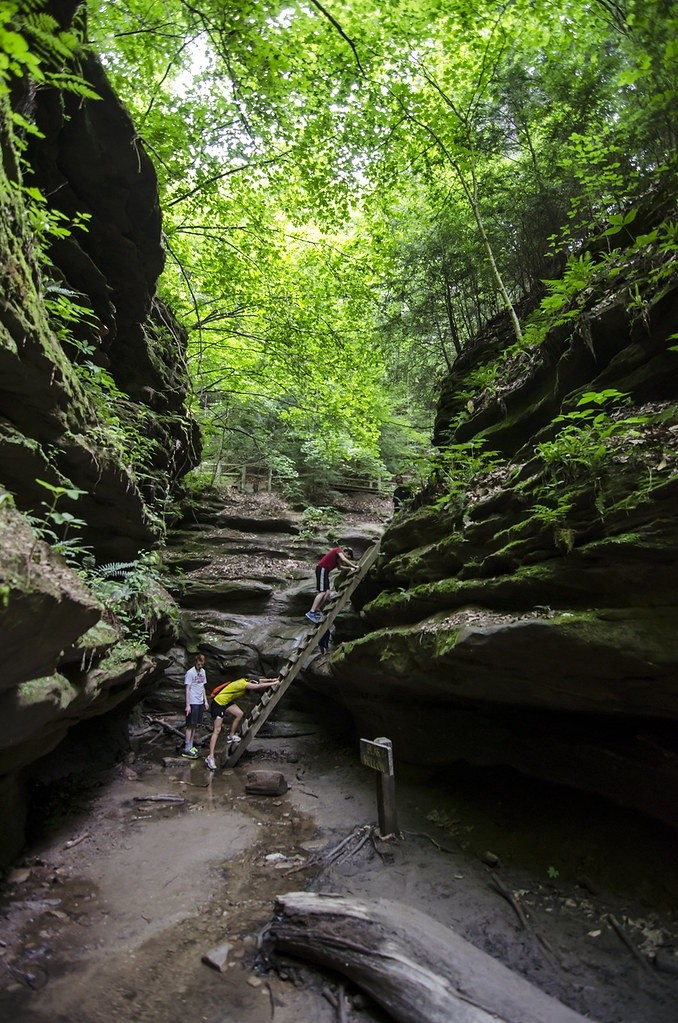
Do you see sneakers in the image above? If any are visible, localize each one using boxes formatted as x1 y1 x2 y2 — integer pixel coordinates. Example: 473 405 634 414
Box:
205 755 217 768
227 735 241 744
315 611 324 618
306 611 319 623
182 749 198 758
191 747 203 757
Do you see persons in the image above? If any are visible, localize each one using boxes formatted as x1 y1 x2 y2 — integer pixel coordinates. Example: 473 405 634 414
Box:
205 676 280 769
313 617 330 653
181 655 209 759
306 547 359 623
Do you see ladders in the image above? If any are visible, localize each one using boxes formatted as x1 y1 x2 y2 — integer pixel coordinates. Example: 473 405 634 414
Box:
219 544 382 767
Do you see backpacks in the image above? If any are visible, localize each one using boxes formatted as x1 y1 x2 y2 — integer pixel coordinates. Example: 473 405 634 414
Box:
211 679 247 698
